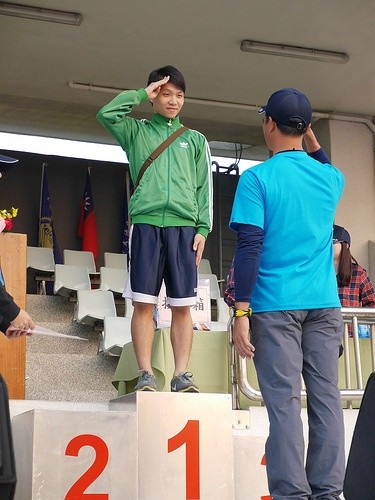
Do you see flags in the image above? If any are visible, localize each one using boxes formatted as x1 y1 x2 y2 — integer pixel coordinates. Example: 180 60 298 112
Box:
77 167 99 264
122 170 130 259
39 162 63 293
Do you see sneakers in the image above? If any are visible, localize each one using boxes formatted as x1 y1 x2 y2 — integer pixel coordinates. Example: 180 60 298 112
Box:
170 372 199 392
134 369 157 392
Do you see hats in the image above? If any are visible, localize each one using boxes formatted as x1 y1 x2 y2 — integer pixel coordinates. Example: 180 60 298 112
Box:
258 88 312 129
332 225 350 248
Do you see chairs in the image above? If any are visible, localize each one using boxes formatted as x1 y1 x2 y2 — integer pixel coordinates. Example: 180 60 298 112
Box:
26 245 231 356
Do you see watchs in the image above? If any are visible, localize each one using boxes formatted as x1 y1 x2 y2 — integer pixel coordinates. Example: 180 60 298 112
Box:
231 308 252 317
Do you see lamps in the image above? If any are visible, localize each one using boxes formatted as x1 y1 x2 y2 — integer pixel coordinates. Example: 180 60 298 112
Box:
0 1 83 27
240 39 350 65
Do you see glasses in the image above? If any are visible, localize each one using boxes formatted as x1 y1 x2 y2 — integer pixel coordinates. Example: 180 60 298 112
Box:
333 239 343 244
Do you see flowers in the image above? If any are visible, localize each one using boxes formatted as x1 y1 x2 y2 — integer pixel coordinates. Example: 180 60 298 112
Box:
0 207 20 233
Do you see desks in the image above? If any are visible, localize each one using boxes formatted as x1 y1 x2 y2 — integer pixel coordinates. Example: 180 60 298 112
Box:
111 328 373 410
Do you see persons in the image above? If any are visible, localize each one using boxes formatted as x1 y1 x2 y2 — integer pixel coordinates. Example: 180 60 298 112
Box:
224 256 236 307
230 88 347 500
0 154 34 500
343 372 375 500
331 224 375 339
95 65 215 392
